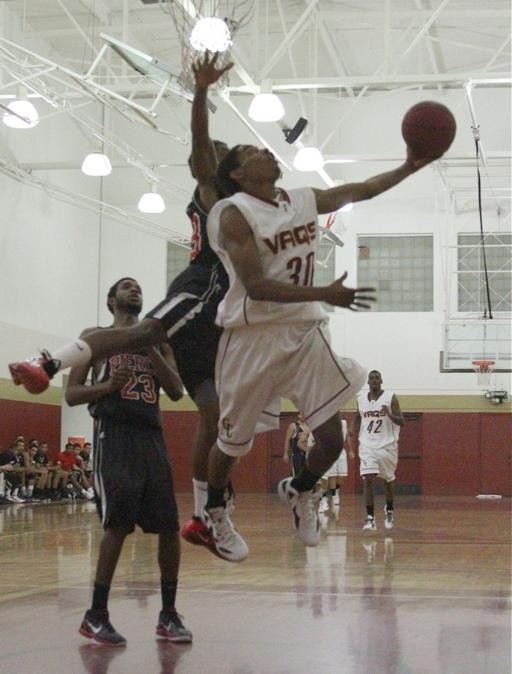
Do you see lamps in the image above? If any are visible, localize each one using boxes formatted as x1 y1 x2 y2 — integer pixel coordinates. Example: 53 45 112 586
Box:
138 184 165 213
246 75 284 123
80 95 112 177
187 0 235 54
2 0 41 129
291 131 324 171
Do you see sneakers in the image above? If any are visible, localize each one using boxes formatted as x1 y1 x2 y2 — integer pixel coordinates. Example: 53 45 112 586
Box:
156 611 192 641
0 491 95 503
79 610 126 646
182 478 393 561
9 356 49 393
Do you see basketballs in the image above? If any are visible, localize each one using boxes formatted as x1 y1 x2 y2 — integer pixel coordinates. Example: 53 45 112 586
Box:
402 102 456 152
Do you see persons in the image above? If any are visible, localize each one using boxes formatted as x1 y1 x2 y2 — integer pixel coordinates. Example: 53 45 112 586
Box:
343 537 400 674
283 409 310 491
352 370 405 532
9 46 279 563
201 139 446 564
318 410 350 512
79 642 193 674
63 275 195 647
1 429 99 507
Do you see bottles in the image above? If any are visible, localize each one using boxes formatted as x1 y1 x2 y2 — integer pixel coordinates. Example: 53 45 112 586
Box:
9 459 63 474
